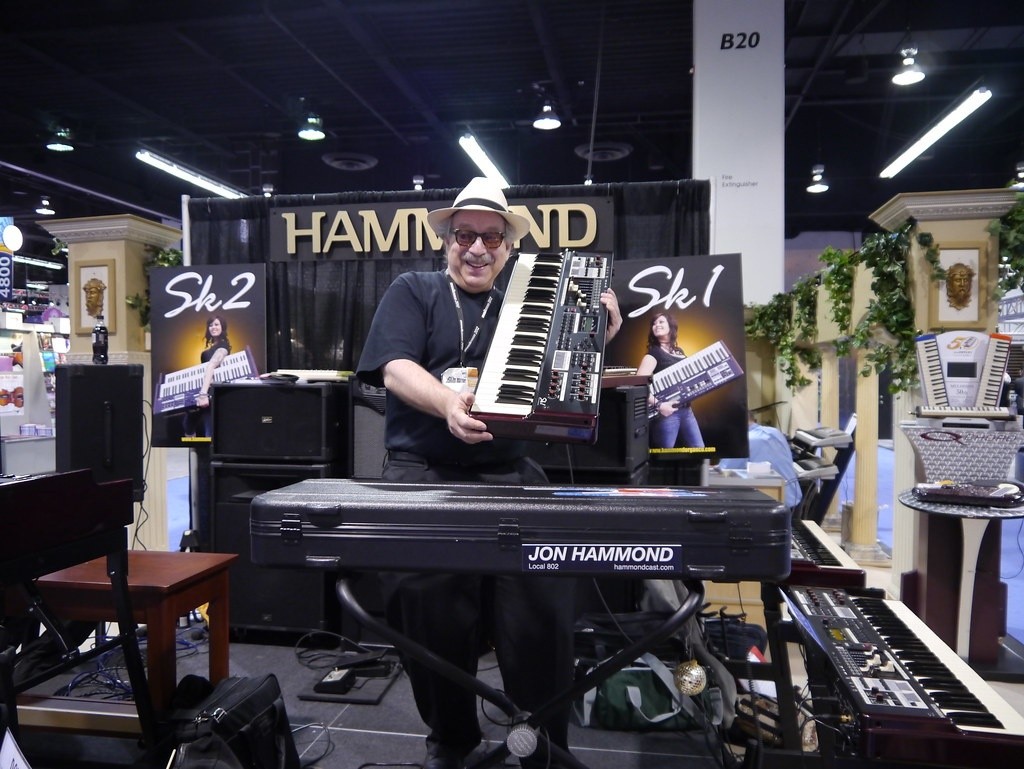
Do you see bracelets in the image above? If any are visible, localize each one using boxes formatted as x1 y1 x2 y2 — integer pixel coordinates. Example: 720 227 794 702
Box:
200 391 209 396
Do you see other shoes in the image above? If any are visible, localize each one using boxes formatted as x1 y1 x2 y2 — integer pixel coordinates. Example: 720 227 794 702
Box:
424 743 466 769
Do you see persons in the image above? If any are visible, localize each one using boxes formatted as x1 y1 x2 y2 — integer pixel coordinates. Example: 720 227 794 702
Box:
197 315 229 438
82 278 106 318
357 176 622 769
945 263 975 310
638 311 705 451
716 412 801 521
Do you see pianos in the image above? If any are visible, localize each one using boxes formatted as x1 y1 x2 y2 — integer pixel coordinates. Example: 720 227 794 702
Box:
771 518 1024 769
792 457 840 519
791 426 856 459
915 333 1017 421
152 348 259 419
645 339 744 420
468 248 615 443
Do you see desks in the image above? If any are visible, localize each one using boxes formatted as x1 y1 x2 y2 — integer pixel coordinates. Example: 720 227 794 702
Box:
31 550 237 712
897 489 1024 667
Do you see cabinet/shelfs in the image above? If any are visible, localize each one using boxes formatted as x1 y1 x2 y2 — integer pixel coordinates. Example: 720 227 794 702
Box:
0 312 71 476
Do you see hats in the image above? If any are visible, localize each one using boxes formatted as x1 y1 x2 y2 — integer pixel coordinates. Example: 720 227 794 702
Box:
427 177 530 243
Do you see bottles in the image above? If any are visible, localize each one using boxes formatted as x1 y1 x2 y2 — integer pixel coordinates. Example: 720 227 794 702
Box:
90 314 110 365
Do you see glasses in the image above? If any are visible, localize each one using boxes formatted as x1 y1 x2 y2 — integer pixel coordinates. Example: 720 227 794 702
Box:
448 227 506 248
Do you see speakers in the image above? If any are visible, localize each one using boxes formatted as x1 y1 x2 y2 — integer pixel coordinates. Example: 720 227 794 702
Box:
523 382 650 617
208 459 349 636
347 373 390 479
55 362 145 502
211 383 351 462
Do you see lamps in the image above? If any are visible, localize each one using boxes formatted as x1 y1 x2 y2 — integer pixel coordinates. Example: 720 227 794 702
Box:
35 194 55 215
46 122 75 151
878 76 992 178
298 111 325 141
136 142 252 198
12 254 65 270
890 28 925 87
806 116 829 193
532 99 562 131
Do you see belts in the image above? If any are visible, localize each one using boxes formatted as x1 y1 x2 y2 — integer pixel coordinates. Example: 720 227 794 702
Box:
387 450 461 467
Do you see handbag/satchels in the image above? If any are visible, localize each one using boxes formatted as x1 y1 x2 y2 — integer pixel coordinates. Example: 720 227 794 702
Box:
170 673 302 769
572 604 767 732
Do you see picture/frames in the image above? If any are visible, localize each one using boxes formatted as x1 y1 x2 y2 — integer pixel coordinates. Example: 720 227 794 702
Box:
73 258 116 335
928 240 988 332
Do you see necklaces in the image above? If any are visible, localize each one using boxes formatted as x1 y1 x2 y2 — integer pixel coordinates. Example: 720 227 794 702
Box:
660 344 669 350
